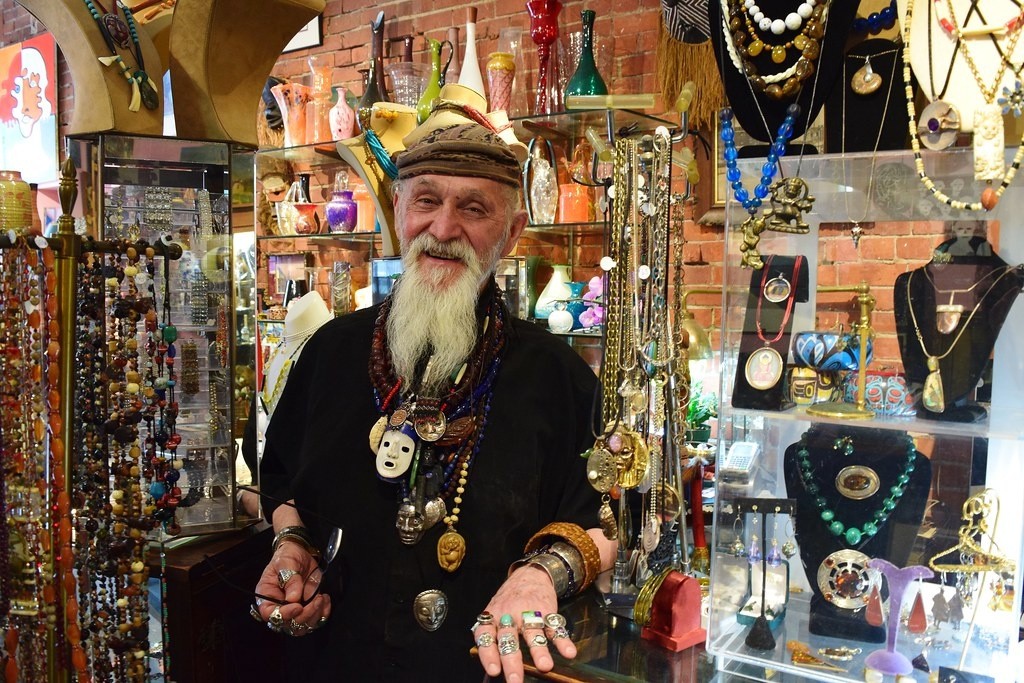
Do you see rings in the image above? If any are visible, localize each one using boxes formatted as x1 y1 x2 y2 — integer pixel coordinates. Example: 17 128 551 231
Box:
249 569 328 639
470 609 571 656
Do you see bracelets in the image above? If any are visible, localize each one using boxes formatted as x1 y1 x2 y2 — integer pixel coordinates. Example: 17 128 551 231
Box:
505 520 603 602
179 279 229 507
271 527 314 551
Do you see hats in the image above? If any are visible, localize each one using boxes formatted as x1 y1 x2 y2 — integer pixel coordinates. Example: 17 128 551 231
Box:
395 123 524 188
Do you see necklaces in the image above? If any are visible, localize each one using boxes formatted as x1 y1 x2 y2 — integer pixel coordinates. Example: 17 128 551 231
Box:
0 232 181 682
586 0 1024 610
253 330 316 426
373 278 513 632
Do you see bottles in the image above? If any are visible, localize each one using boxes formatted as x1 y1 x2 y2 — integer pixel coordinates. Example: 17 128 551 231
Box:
324 190 358 233
331 261 352 316
290 174 320 236
485 51 516 119
268 6 488 156
0 170 42 237
564 9 609 112
525 255 589 334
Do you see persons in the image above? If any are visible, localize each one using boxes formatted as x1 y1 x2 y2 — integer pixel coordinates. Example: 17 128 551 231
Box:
233 123 648 683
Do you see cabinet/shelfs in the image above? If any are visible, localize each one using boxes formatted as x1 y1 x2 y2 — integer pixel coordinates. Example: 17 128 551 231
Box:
704 145 1024 683
97 131 238 539
252 108 678 521
